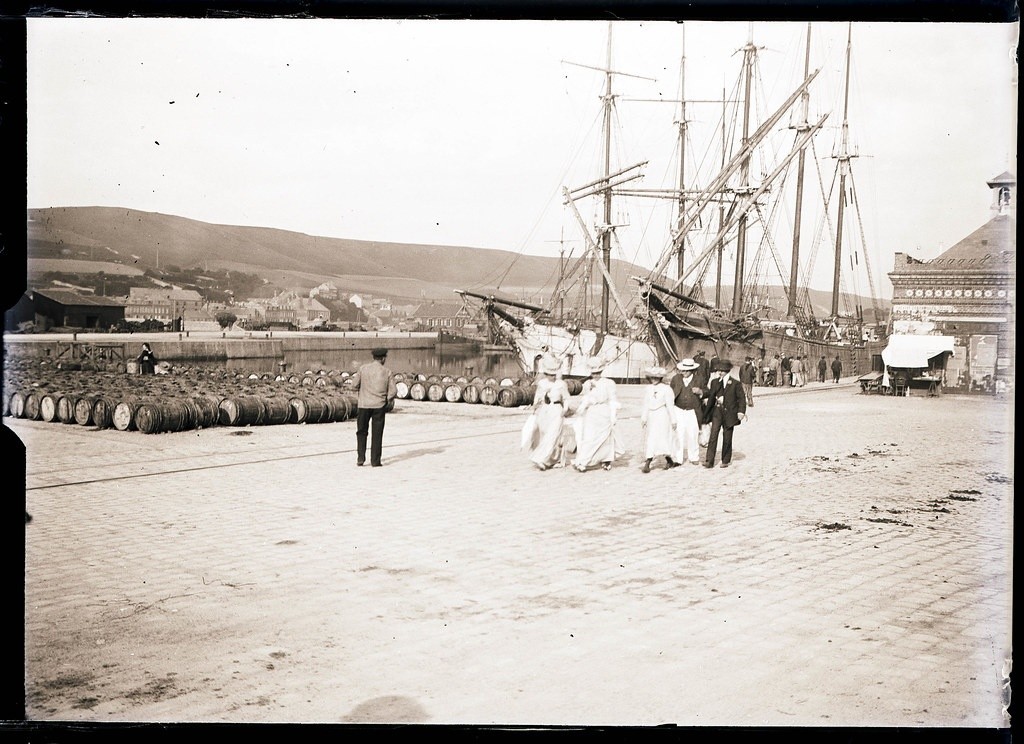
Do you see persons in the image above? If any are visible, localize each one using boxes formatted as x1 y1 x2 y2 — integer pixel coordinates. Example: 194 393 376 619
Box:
670 358 710 466
691 350 721 389
572 357 619 472
751 352 810 388
831 355 842 384
640 366 684 473
818 356 827 383
521 354 573 471
351 347 397 467
739 356 756 407
133 342 158 375
701 359 747 469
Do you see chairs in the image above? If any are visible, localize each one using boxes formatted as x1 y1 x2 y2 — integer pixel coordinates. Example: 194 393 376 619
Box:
895 378 907 396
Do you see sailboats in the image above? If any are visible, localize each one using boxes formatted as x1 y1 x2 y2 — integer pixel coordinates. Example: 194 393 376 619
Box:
453 20 888 386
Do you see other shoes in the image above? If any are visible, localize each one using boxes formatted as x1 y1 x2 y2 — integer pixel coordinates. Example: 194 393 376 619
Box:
372 463 382 466
643 466 650 473
664 461 673 469
692 461 699 465
357 460 364 465
702 461 713 468
672 463 679 467
602 462 611 470
720 464 727 467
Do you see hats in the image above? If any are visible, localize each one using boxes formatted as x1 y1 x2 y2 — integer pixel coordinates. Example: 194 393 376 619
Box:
588 357 605 373
371 348 388 357
774 355 780 358
677 358 700 371
543 358 559 375
644 366 666 378
745 357 751 361
717 360 733 371
700 352 705 356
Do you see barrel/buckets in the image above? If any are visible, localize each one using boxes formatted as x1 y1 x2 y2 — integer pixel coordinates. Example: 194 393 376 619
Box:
1 356 583 434
